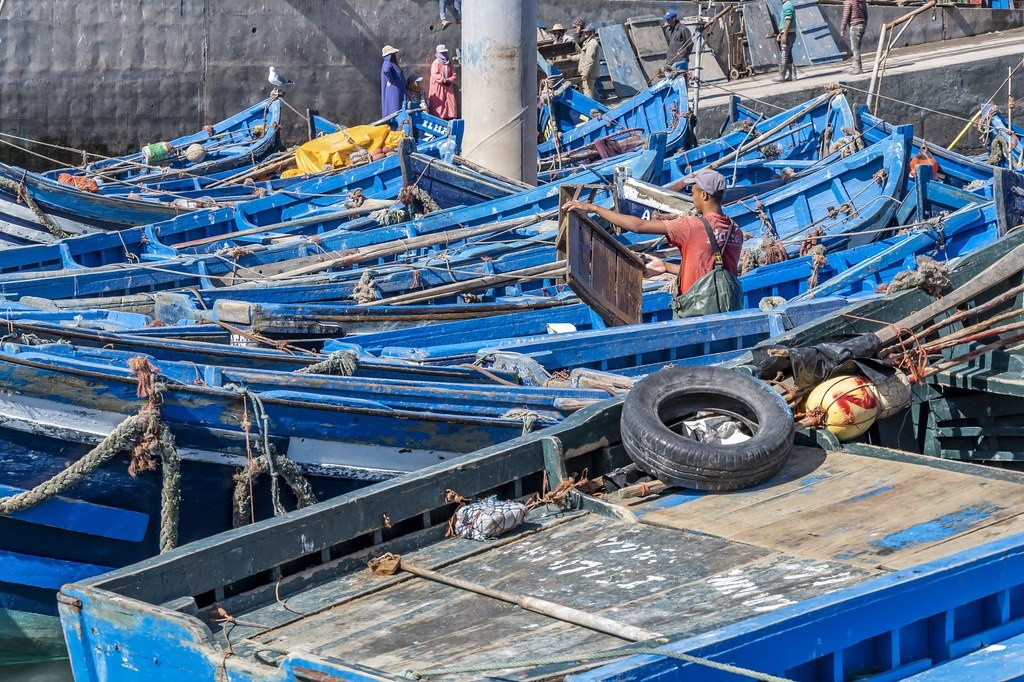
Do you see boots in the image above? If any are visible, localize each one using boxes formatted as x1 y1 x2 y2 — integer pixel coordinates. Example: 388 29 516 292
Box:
848 50 863 74
772 63 788 82
786 63 797 81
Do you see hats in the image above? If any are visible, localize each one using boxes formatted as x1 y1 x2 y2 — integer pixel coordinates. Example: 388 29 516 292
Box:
381 45 400 57
436 44 449 52
663 11 677 20
549 24 567 34
572 18 586 29
581 25 594 33
683 169 725 199
406 75 423 83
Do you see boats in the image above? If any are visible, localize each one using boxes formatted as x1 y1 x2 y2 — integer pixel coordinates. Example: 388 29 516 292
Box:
0 59 1024 660
54 222 1024 682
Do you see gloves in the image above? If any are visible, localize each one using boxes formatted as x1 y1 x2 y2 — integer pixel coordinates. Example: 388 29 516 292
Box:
444 81 451 87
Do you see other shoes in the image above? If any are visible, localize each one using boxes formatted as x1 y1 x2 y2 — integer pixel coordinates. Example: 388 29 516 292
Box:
441 20 451 30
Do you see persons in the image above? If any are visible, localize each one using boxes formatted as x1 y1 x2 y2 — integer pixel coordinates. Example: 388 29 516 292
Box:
662 11 694 65
562 170 743 292
439 0 462 30
427 45 457 119
772 0 797 82
571 25 600 100
841 0 868 74
549 23 582 59
572 19 587 48
381 45 428 118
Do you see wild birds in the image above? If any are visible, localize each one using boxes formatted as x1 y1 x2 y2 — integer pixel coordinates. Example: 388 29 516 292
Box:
268 66 296 87
